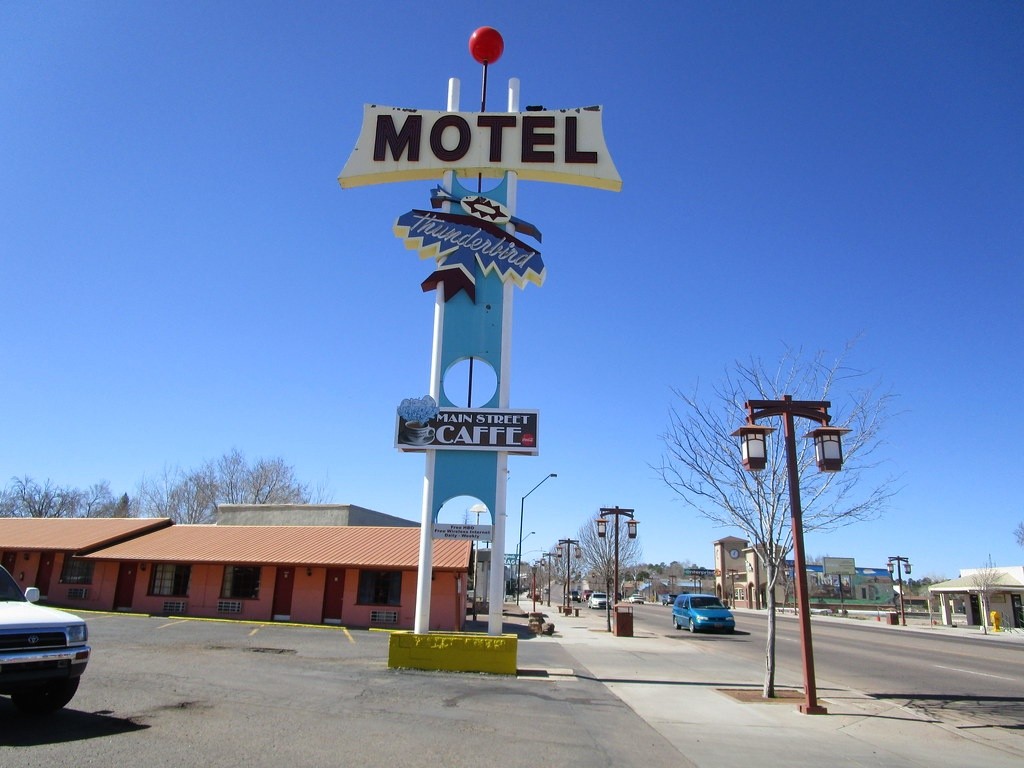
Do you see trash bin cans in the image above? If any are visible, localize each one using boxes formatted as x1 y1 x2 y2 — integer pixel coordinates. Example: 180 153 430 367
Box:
989 610 1003 633
613 603 634 637
886 608 898 624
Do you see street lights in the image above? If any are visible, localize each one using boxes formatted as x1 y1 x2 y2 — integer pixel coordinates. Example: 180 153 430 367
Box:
515 473 558 607
540 551 561 608
887 555 912 626
726 568 740 609
514 531 535 593
784 564 798 616
731 394 854 715
556 538 582 617
595 506 641 638
688 575 700 594
531 564 538 611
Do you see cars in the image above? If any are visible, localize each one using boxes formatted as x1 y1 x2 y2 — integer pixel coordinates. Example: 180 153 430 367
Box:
588 593 612 609
662 594 678 606
0 563 91 714
629 594 645 605
567 590 582 604
672 593 736 634
584 589 594 601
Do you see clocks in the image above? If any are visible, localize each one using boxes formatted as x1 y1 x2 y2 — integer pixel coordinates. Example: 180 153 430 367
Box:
730 549 739 559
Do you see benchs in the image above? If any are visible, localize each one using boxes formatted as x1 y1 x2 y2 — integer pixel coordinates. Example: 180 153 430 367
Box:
574 608 582 617
557 606 572 616
